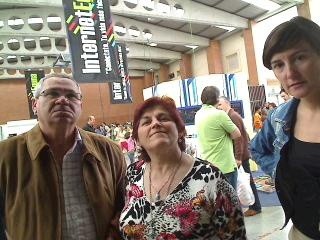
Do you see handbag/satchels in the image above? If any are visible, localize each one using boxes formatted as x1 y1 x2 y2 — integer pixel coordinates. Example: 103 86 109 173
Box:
237 164 255 207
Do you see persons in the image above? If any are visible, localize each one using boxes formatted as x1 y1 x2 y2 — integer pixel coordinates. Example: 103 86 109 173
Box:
109 94 248 240
82 116 97 133
194 86 242 193
279 88 292 101
249 16 320 240
97 121 141 166
262 102 277 122
216 96 263 217
0 73 129 239
254 106 262 134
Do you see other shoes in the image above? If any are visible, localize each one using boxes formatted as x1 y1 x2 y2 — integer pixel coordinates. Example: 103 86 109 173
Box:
244 209 261 217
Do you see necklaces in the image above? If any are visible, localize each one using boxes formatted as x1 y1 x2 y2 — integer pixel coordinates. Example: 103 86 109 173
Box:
149 150 185 237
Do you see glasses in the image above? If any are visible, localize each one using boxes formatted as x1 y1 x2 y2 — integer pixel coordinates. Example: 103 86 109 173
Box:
35 87 83 101
